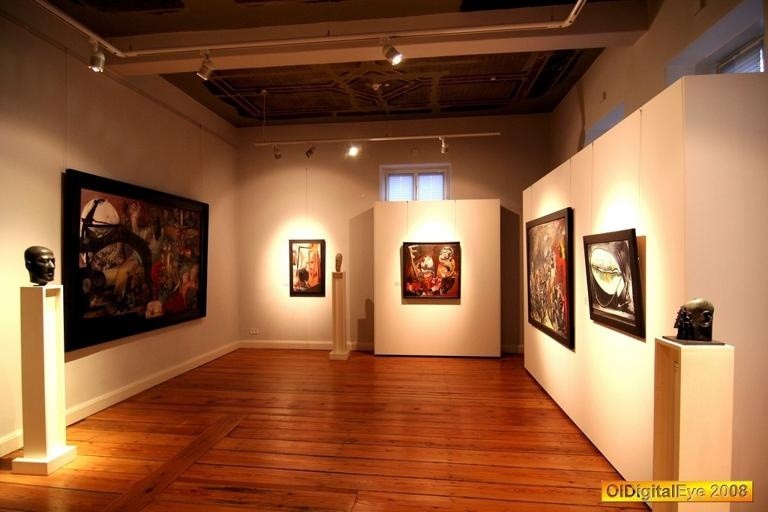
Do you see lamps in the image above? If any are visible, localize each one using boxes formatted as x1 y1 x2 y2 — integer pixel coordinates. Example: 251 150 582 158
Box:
304 142 316 160
378 35 403 66
272 143 282 159
196 47 215 81
85 40 107 72
437 134 450 154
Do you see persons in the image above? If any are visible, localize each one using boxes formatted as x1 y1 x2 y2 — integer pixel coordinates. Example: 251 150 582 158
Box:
24 246 56 285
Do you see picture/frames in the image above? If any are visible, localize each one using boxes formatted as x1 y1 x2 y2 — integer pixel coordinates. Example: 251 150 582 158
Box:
582 228 646 339
289 239 325 297
401 241 461 300
61 169 209 353
525 207 578 350
